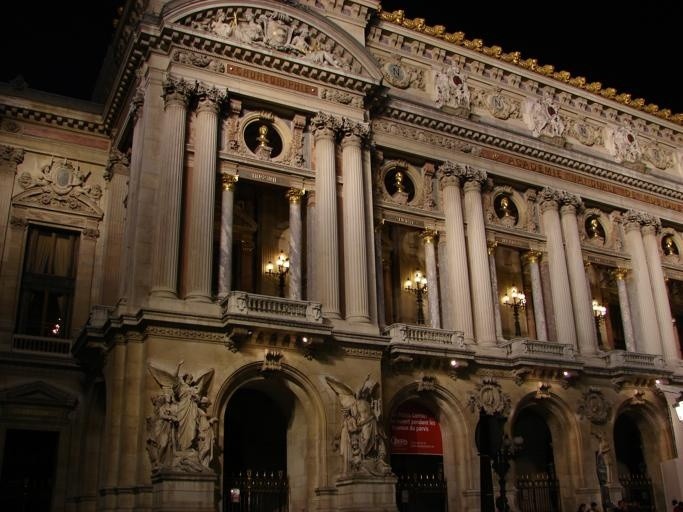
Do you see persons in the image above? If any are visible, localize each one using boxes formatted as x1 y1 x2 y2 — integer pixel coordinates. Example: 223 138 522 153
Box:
226 10 265 43
393 172 406 192
330 370 394 484
665 238 675 254
256 125 270 142
210 11 226 36
590 219 600 237
499 197 512 217
145 357 219 474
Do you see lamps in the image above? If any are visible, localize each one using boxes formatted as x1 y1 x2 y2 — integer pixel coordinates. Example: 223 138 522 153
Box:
593 298 606 348
502 285 527 337
672 390 683 421
266 249 289 298
405 267 427 324
